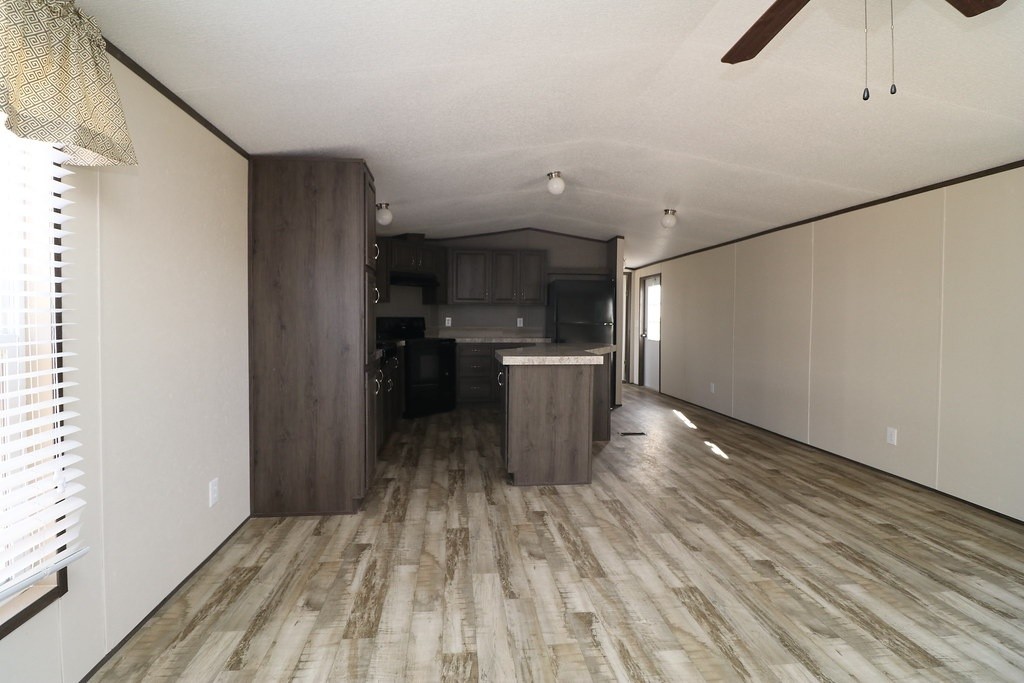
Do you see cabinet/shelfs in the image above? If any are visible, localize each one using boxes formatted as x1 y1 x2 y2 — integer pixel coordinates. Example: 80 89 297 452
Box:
492 342 611 484
248 155 490 516
492 249 546 305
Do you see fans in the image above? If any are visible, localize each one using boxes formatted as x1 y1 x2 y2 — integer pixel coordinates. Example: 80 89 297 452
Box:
720 0 1008 65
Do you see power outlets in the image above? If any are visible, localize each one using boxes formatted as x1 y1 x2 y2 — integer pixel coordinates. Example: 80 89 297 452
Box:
517 318 523 327
445 317 452 326
887 428 896 445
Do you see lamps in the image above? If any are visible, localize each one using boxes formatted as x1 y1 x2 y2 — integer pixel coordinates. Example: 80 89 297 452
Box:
547 171 565 195
376 203 392 225
661 209 677 228
